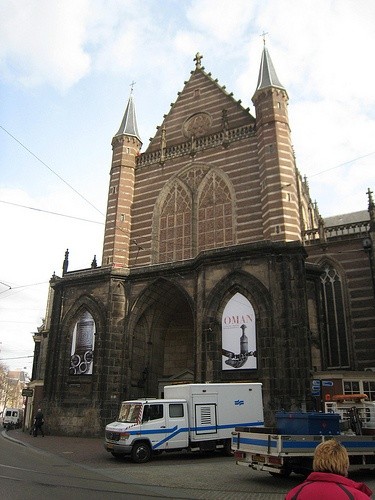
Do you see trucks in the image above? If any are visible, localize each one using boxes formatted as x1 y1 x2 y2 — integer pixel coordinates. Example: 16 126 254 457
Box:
231 394 375 479
104 383 265 463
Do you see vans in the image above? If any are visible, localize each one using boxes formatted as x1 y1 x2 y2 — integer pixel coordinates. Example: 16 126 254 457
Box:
3 408 23 427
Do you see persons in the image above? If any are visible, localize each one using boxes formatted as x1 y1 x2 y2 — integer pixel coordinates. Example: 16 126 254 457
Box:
283 440 375 500
33 409 44 437
